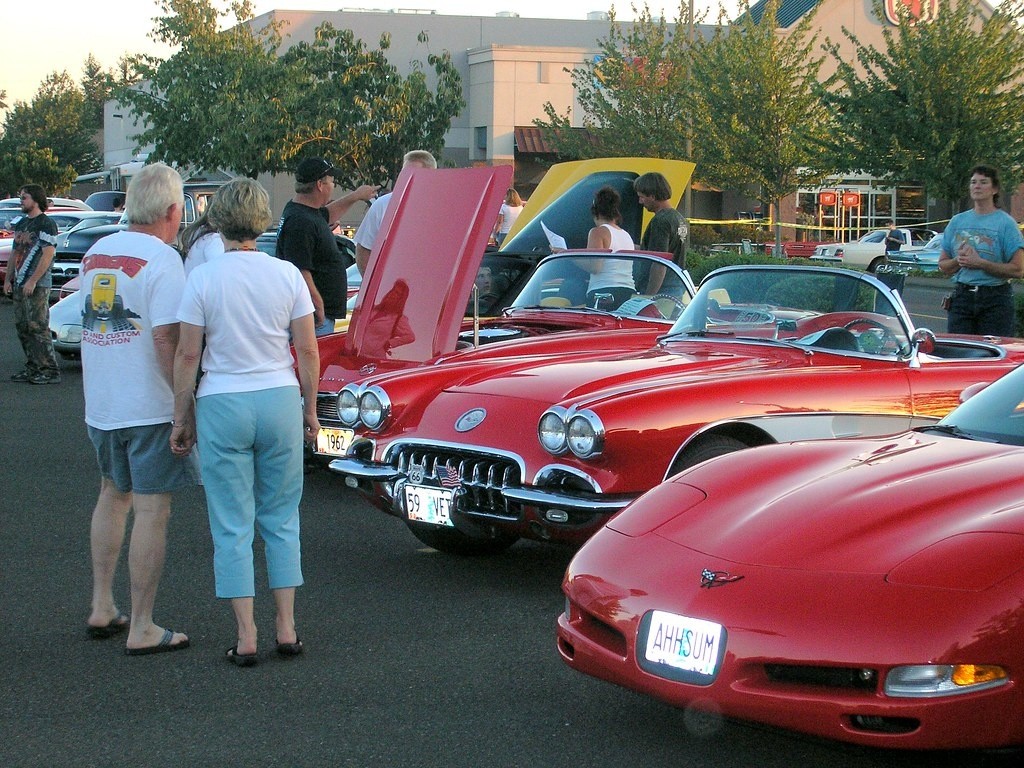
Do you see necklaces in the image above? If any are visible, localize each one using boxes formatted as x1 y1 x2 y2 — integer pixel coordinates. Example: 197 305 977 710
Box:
225 247 261 252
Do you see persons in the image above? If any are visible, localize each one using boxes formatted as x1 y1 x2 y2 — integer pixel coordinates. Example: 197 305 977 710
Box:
548 187 637 312
81 164 195 655
353 151 437 278
885 220 905 252
939 166 1024 336
4 186 61 383
361 280 415 358
113 197 122 224
170 179 320 664
275 159 382 336
494 188 523 246
634 172 689 298
181 195 225 485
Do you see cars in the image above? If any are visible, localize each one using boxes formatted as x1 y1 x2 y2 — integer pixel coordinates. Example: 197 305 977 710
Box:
808 227 937 273
0 154 906 362
875 232 944 274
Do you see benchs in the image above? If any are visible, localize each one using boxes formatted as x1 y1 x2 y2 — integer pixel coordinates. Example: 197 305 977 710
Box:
846 336 994 364
673 303 772 323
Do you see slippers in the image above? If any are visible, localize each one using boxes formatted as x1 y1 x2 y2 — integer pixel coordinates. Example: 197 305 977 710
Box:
86 611 131 640
225 646 257 668
275 633 303 654
124 628 190 656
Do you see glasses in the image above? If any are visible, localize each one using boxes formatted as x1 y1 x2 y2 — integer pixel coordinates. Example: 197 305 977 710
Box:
19 195 31 201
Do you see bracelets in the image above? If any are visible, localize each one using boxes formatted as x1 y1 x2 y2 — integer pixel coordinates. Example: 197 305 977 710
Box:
171 420 188 427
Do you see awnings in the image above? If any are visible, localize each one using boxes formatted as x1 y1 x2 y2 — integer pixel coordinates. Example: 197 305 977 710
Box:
515 126 683 154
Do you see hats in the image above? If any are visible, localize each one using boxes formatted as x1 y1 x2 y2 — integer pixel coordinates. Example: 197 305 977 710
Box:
295 156 343 183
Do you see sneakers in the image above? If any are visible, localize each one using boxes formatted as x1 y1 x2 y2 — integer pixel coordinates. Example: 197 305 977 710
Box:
30 372 61 383
10 366 36 382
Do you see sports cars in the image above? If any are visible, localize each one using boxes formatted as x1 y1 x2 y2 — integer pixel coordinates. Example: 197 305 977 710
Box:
556 362 1024 747
286 162 821 475
326 264 1023 564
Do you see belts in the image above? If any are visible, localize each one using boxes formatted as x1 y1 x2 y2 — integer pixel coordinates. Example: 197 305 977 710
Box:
957 283 1010 292
